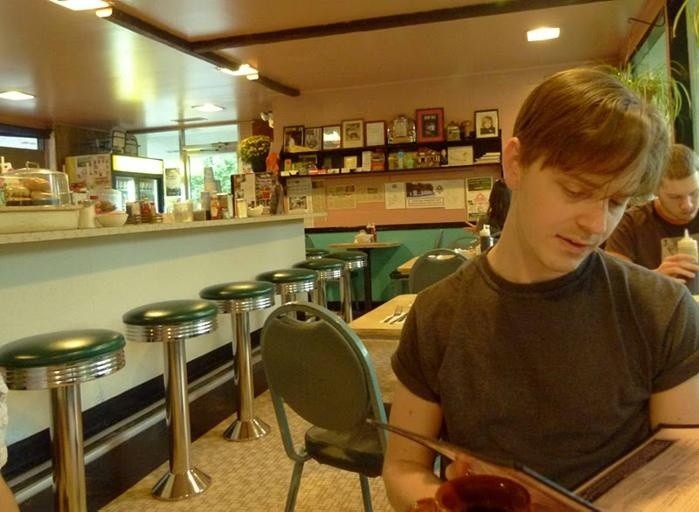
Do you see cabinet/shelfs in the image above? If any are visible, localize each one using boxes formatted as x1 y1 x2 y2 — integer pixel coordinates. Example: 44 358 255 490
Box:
276 129 503 195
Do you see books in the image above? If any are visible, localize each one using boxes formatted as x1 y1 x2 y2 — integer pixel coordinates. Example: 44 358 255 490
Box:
375 423 699 512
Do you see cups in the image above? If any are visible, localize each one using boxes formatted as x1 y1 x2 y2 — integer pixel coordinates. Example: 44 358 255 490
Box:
406 473 530 511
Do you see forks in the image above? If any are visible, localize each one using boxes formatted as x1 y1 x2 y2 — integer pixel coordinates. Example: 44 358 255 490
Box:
380 305 403 323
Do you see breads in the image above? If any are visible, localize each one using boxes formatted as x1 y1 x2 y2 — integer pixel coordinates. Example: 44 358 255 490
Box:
7 177 54 200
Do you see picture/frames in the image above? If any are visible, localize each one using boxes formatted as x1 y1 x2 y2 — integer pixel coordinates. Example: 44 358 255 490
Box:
416 108 444 143
283 119 386 153
474 109 499 139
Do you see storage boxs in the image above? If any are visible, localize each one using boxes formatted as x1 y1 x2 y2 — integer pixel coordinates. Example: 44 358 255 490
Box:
448 145 475 165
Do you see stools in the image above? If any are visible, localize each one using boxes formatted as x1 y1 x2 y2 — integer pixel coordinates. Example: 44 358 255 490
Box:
195 280 277 443
293 258 345 310
324 249 368 323
120 298 218 503
306 250 329 305
1 325 128 512
254 266 318 305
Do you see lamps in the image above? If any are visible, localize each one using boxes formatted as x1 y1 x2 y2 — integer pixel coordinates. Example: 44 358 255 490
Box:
232 62 259 81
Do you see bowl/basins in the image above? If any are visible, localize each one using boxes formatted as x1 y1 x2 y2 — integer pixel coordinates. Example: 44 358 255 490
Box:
95 214 129 228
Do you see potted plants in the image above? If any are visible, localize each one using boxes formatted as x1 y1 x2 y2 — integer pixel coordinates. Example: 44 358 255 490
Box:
237 135 272 172
594 61 692 124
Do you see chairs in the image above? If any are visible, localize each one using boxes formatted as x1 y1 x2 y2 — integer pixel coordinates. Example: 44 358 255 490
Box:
261 301 442 512
407 248 469 294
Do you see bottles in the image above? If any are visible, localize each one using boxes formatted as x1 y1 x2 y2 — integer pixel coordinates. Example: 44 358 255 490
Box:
205 192 248 220
126 198 156 224
480 224 490 253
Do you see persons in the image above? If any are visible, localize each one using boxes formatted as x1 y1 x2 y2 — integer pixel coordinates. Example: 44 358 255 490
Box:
381 68 699 512
603 143 699 295
480 116 496 135
463 180 512 238
